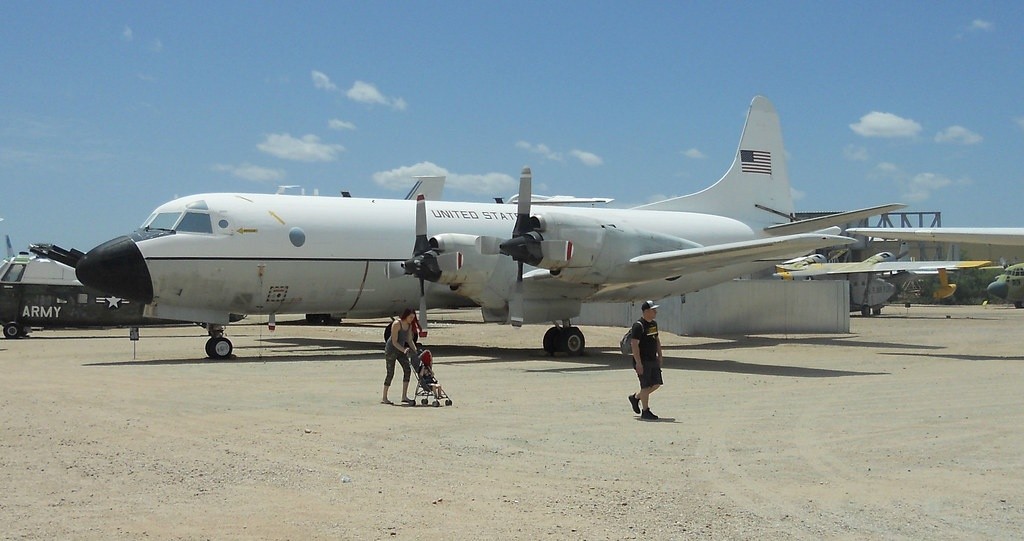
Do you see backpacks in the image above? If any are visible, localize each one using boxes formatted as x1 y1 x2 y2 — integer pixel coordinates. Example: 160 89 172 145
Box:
620 321 644 356
384 321 393 342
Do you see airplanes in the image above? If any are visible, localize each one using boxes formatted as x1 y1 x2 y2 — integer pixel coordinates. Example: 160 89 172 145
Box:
1 95 1024 358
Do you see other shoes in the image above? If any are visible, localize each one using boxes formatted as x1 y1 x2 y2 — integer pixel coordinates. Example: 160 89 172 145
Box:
401 399 416 404
380 401 393 404
641 408 658 419
628 392 640 414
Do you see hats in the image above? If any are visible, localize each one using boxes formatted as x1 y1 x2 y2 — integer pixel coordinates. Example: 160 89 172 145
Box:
641 300 660 311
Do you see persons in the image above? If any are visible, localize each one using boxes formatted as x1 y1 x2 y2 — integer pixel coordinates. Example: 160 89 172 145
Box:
404 305 422 348
419 364 444 398
628 300 663 420
381 307 418 404
